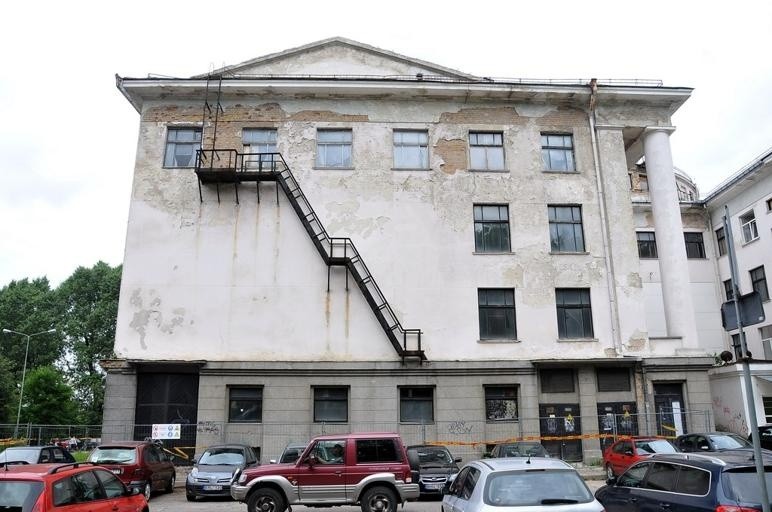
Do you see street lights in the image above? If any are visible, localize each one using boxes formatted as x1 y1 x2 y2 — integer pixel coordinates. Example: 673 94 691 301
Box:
4 325 61 443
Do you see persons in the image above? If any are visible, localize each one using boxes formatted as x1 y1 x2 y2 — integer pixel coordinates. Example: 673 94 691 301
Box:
315 444 344 464
62 435 81 451
